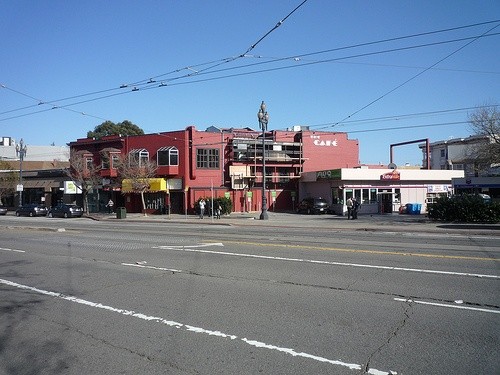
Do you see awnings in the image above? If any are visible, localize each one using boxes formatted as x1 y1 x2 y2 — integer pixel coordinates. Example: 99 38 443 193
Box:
121 178 167 193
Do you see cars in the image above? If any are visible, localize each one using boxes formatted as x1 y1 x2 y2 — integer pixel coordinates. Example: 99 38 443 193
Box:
296 195 329 215
15 203 49 217
47 204 83 219
0 202 8 215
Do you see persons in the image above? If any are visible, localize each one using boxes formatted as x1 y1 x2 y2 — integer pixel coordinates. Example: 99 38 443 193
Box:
351 198 359 220
346 197 353 220
216 202 223 219
199 197 206 219
105 199 115 215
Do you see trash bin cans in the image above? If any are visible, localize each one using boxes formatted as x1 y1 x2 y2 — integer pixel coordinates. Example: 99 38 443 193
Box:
117 207 126 219
405 203 422 215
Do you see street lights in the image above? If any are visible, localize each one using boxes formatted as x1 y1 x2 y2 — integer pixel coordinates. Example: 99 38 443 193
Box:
257 100 270 220
14 138 27 208
